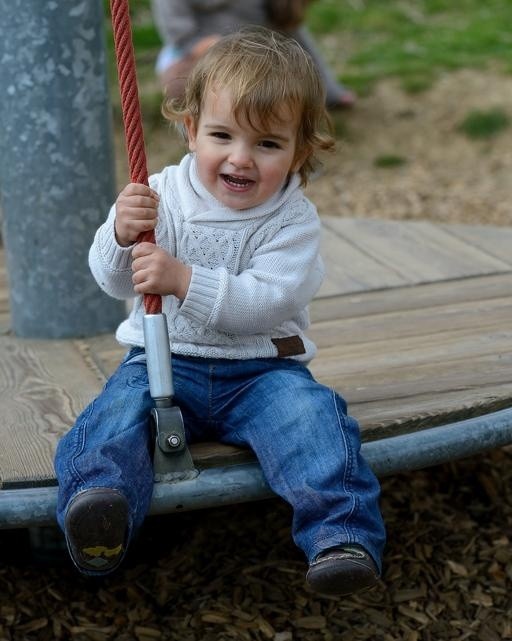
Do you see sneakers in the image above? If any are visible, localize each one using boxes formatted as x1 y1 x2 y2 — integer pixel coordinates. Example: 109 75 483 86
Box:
307 542 375 600
65 486 134 579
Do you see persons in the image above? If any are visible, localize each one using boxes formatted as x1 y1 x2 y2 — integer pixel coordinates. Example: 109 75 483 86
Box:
153 0 356 118
53 25 387 599
